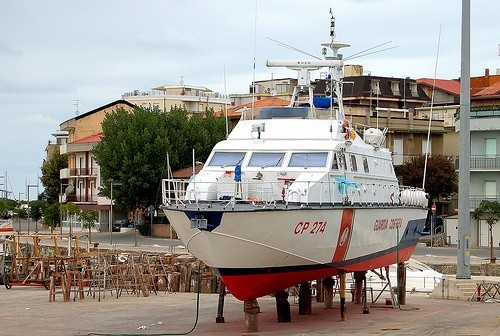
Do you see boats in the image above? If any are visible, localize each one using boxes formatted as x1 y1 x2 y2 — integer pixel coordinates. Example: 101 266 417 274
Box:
159 7 430 303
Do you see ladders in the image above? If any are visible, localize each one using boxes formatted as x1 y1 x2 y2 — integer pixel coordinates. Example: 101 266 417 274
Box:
67 251 176 302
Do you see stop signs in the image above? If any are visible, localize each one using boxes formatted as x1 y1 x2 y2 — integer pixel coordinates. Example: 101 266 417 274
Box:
432 205 436 213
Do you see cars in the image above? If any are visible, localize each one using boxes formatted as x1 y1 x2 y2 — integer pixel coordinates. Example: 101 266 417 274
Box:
311 259 443 293
421 216 444 235
112 219 134 232
